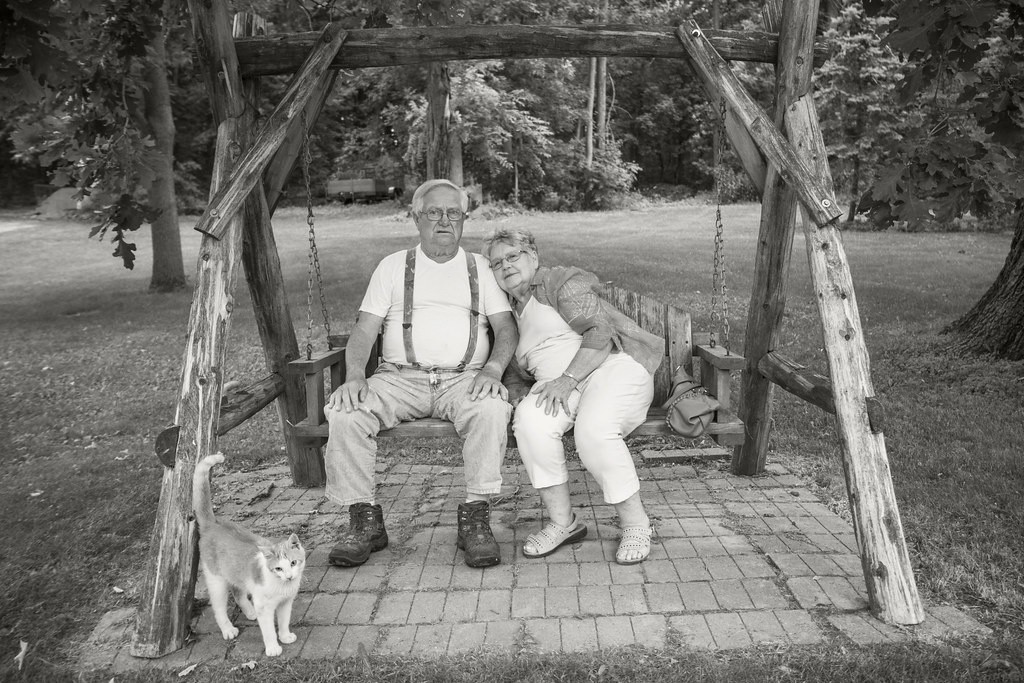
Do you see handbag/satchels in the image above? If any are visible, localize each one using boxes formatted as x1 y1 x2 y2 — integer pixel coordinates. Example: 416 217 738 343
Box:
661 362 720 437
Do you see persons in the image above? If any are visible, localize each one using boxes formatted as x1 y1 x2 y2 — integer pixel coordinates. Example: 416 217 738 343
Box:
481 224 666 564
324 179 520 567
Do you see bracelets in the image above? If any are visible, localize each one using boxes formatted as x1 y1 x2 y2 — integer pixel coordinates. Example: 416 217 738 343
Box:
562 372 580 381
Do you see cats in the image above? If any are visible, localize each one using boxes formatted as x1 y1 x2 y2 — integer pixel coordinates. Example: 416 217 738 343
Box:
192 454 307 657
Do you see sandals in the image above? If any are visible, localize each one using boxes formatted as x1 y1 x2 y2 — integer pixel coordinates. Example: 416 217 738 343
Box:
615 524 652 564
523 512 588 559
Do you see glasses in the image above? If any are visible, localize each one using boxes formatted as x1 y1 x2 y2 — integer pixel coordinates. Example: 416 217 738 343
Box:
488 250 528 271
419 208 468 221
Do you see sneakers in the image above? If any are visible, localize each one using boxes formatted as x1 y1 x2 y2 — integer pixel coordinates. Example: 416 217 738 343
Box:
456 500 501 567
328 501 388 567
